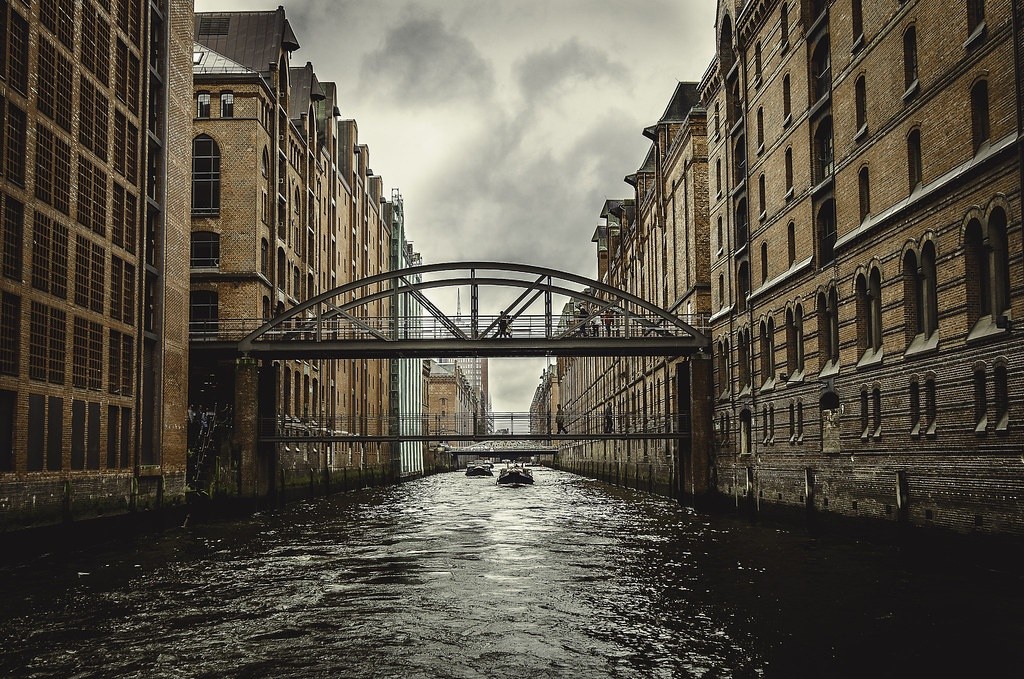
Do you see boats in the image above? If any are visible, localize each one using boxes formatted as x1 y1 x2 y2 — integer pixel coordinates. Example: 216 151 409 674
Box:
465 460 491 476
496 460 535 485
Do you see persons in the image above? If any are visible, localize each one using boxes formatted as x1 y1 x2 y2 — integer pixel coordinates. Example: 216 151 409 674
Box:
603 402 613 434
497 311 513 338
556 404 569 434
577 308 614 337
